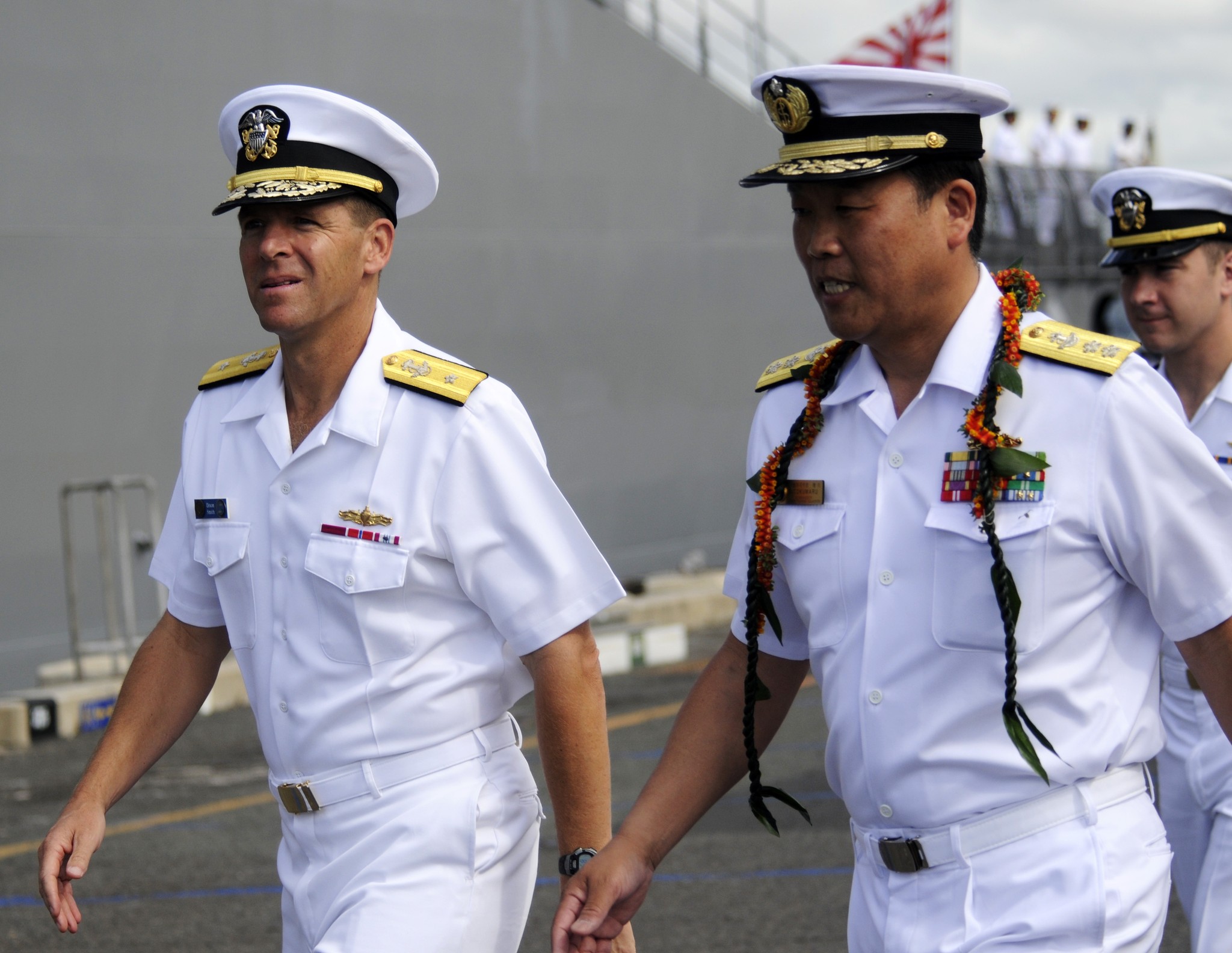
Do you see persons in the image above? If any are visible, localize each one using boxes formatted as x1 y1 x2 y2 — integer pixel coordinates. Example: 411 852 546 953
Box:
1075 166 1232 953
548 62 1232 953
31 83 639 953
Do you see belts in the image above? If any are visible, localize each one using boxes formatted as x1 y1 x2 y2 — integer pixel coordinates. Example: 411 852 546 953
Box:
1161 655 1202 689
271 714 516 815
854 763 1149 872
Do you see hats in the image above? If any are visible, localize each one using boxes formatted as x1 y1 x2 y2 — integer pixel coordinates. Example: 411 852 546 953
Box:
212 84 439 229
1088 166 1232 268
736 64 1009 188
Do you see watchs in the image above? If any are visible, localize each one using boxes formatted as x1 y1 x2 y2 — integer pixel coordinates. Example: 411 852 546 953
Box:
558 846 598 876
